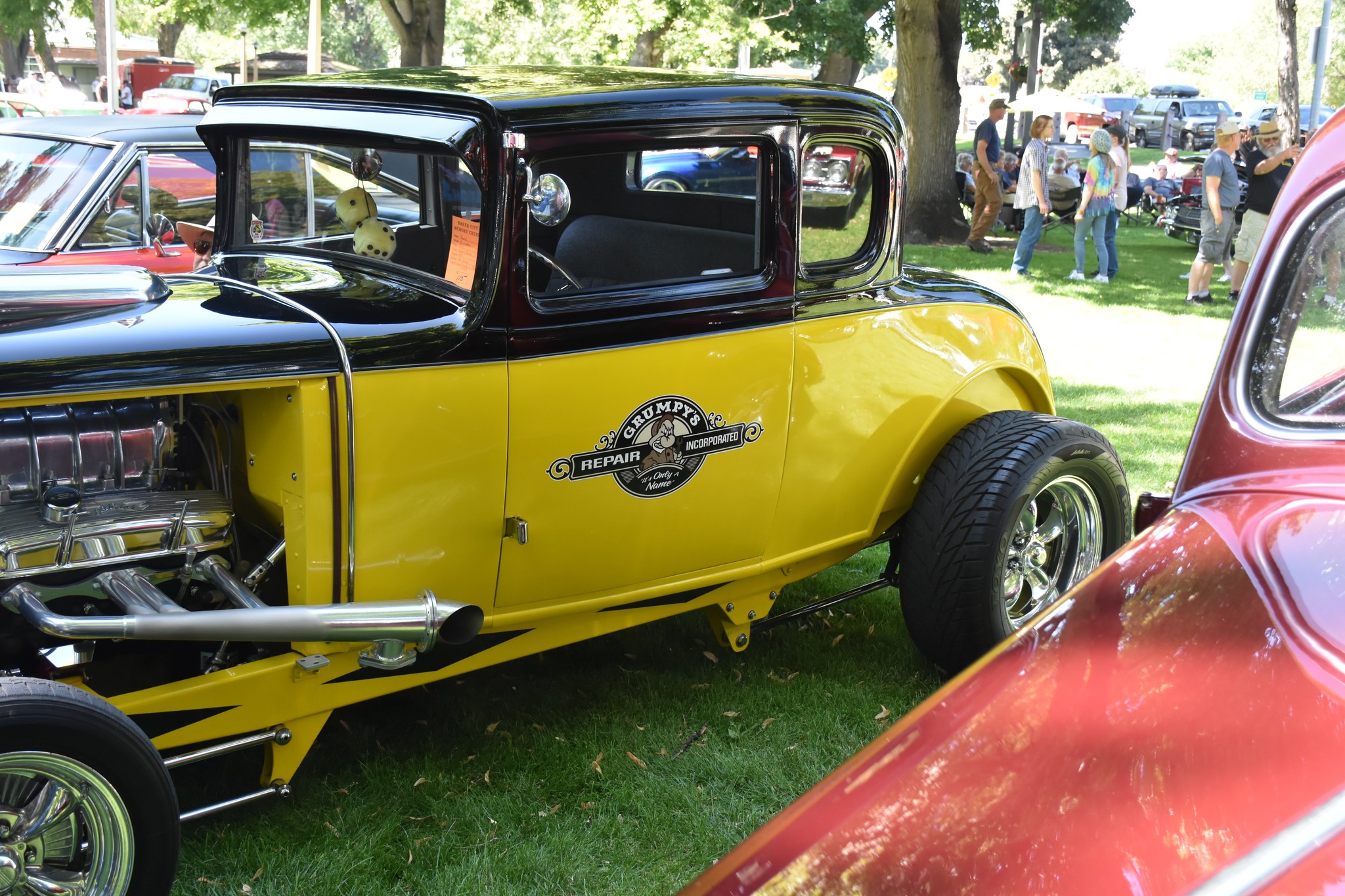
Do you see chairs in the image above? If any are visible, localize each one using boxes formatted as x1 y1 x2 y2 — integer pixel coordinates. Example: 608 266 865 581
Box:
1042 186 1081 238
103 184 178 243
1121 187 1143 226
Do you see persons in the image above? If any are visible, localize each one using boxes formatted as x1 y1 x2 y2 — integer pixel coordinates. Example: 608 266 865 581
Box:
1144 124 1319 281
1184 120 1240 307
957 144 1143 234
176 172 290 271
1230 122 1305 303
91 75 134 110
1010 115 1055 280
965 99 1011 253
1062 130 1115 284
1089 123 1130 278
0 70 88 101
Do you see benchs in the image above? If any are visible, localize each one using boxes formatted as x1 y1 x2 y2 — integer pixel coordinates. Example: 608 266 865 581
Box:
546 214 754 292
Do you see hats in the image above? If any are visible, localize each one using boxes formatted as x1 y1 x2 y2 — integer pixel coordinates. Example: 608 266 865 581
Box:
1016 149 1025 158
1165 148 1177 157
101 75 107 81
1092 129 1112 154
1216 120 1247 136
1103 124 1126 139
989 99 1012 110
1251 120 1287 140
123 80 128 84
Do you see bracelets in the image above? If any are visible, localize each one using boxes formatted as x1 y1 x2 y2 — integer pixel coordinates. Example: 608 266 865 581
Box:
1076 208 1084 215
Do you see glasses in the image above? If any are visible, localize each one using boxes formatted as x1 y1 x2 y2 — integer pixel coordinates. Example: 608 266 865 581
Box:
1047 126 1054 128
1196 167 1202 171
968 162 974 165
1264 136 1278 143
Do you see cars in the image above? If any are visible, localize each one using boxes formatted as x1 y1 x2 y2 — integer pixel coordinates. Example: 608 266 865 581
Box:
1247 103 1340 136
2 63 1144 895
675 107 1345 896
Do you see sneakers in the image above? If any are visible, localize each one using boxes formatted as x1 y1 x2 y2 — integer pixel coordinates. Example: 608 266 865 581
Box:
1197 293 1218 307
1087 273 1109 284
1063 269 1085 281
1179 272 1190 279
1183 295 1203 307
1217 272 1232 282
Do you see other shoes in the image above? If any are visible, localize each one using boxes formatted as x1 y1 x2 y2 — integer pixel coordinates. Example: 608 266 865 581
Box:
1091 271 1099 275
1065 215 1075 224
1228 290 1239 301
1006 223 1015 232
965 237 993 254
1042 215 1054 224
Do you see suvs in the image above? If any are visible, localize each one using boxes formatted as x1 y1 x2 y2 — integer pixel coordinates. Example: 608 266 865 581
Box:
1064 83 1238 153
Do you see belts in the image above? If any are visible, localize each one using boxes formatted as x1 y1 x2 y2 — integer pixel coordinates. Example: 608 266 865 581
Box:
1202 205 1237 212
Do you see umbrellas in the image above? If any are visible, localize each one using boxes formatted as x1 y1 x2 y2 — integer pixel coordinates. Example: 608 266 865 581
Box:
1005 85 1107 119
